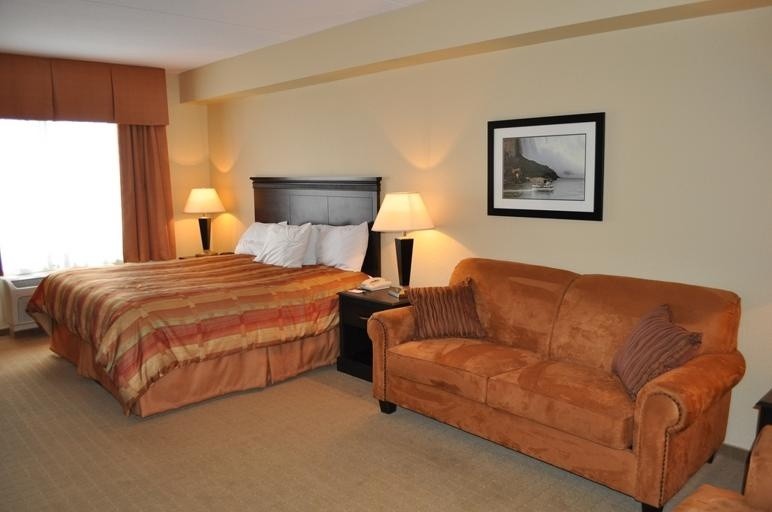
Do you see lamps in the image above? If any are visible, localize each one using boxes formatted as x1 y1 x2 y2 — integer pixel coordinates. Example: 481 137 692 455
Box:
183 188 225 256
370 193 435 298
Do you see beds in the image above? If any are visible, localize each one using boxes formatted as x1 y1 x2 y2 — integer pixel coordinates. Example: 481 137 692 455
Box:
25 177 382 419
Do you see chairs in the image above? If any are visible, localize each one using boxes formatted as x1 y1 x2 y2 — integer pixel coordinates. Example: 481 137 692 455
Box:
671 424 772 512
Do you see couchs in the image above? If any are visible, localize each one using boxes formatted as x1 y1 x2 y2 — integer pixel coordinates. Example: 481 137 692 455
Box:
366 258 746 512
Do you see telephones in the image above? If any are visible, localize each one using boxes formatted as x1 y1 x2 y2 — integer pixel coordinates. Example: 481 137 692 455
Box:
362 277 390 292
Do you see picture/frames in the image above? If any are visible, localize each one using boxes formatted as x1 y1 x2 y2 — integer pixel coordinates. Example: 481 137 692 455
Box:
487 113 605 221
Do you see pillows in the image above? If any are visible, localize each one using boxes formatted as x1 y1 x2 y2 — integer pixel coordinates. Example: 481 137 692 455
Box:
404 276 487 342
234 221 369 272
612 304 703 402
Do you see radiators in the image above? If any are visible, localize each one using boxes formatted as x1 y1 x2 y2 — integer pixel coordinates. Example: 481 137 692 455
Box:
1 276 47 334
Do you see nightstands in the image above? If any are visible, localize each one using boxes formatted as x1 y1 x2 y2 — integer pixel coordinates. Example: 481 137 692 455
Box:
336 287 409 382
